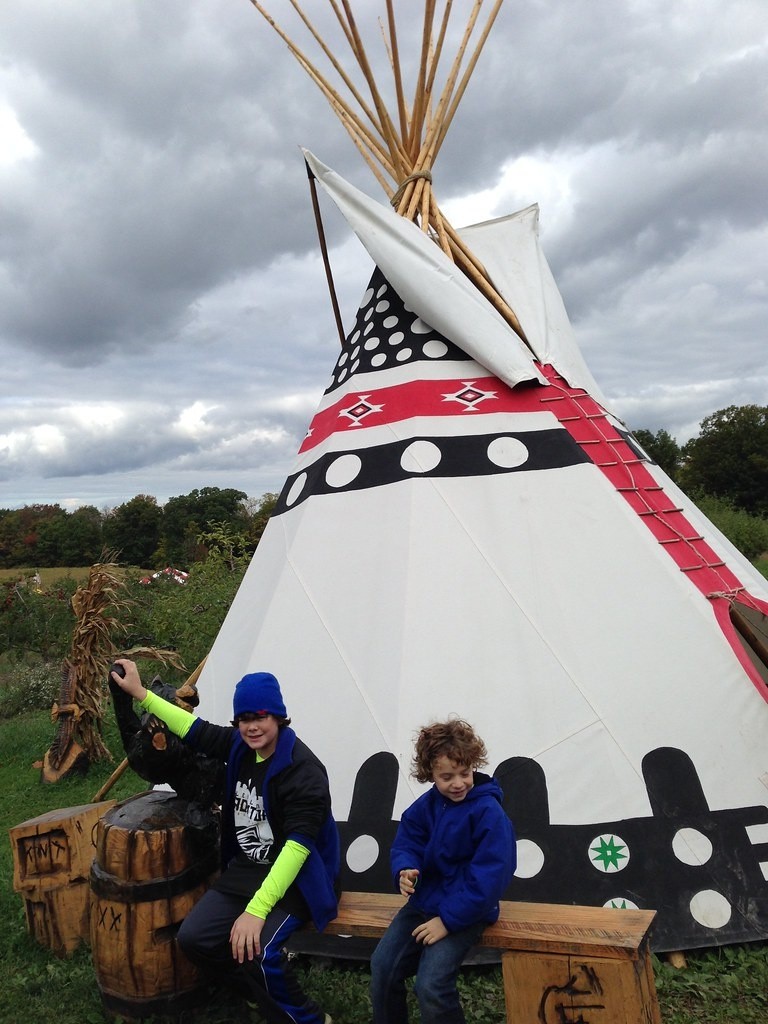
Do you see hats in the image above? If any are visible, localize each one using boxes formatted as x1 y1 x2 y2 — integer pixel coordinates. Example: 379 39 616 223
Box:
233 672 287 717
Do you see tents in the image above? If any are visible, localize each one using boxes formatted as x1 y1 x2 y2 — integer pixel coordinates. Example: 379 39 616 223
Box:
154 145 768 962
132 566 190 585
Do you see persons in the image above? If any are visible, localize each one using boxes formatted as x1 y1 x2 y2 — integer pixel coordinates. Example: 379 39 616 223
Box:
371 721 517 1024
18 575 27 587
112 659 342 1024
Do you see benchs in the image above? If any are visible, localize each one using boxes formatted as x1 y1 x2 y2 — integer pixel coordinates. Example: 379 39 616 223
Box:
291 891 664 1024
